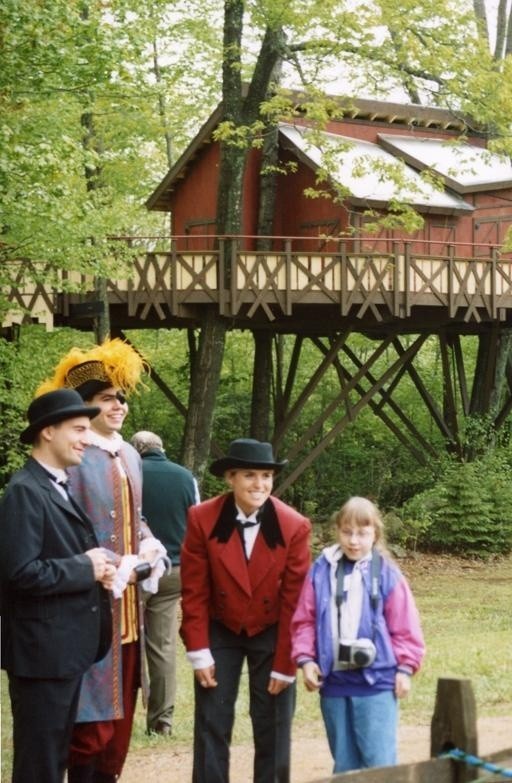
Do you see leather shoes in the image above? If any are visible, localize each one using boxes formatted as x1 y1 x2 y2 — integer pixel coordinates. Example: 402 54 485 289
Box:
145 722 172 737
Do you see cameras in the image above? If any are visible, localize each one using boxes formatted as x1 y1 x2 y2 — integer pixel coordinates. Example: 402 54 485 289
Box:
338 638 376 667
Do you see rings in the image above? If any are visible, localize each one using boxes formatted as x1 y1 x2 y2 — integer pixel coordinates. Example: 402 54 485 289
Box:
201 680 207 685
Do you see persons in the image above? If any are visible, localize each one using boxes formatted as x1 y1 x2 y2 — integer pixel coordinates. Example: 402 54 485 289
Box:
176 437 311 783
0 389 119 782
130 427 200 736
289 495 427 775
67 382 173 783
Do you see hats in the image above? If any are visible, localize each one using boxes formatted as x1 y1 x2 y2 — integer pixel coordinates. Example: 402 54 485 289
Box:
66 359 122 401
19 389 101 445
207 438 290 476
